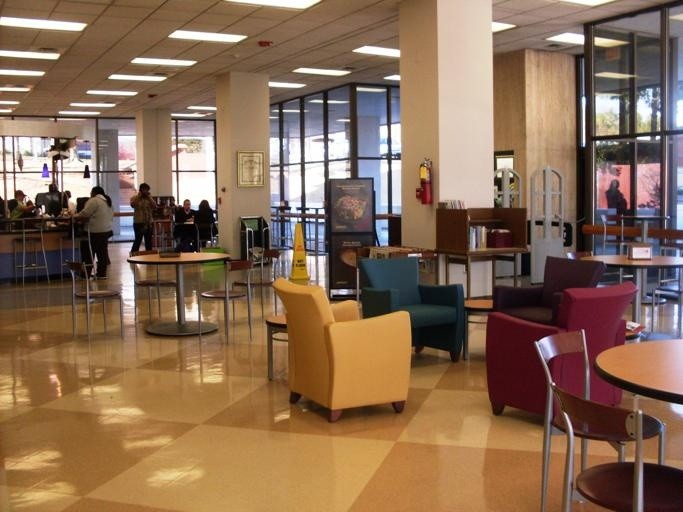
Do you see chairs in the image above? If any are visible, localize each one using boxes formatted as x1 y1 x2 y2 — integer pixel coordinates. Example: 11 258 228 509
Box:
198 261 252 339
134 250 177 322
645 244 681 336
271 207 293 247
64 259 126 344
297 208 310 251
10 217 50 289
57 215 97 283
548 381 682 512
532 328 666 512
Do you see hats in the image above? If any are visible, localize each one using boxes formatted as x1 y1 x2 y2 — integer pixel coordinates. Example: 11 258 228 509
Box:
15 190 27 197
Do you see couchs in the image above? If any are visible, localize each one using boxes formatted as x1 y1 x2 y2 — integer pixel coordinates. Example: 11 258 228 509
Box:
487 280 638 416
493 256 608 324
357 257 464 362
273 277 411 422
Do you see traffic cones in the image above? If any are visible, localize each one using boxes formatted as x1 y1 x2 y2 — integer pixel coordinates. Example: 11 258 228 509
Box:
287 221 311 282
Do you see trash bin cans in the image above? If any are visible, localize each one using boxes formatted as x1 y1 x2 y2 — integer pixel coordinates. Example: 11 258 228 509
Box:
626 320 642 343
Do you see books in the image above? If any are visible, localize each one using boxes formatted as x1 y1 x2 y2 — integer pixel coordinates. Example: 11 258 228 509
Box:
438 199 488 250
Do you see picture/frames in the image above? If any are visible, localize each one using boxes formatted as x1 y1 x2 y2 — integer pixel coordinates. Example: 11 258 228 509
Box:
236 151 265 189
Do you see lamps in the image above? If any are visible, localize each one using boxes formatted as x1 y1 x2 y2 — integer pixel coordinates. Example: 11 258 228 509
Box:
82 140 90 179
40 136 49 178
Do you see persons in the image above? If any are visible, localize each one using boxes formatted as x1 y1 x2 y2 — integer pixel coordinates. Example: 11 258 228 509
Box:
9 184 74 229
606 179 627 215
74 186 113 281
130 183 156 252
184 200 218 250
175 199 196 224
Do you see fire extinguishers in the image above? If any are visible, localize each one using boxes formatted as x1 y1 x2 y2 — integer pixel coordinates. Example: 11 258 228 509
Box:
419 158 433 205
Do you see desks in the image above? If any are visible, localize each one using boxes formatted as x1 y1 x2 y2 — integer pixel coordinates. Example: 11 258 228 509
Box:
594 338 683 466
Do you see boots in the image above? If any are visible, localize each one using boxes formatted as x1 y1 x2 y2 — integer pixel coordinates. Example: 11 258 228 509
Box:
94 261 107 280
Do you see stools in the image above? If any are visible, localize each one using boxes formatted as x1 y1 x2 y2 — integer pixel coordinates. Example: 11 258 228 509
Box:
266 315 288 380
